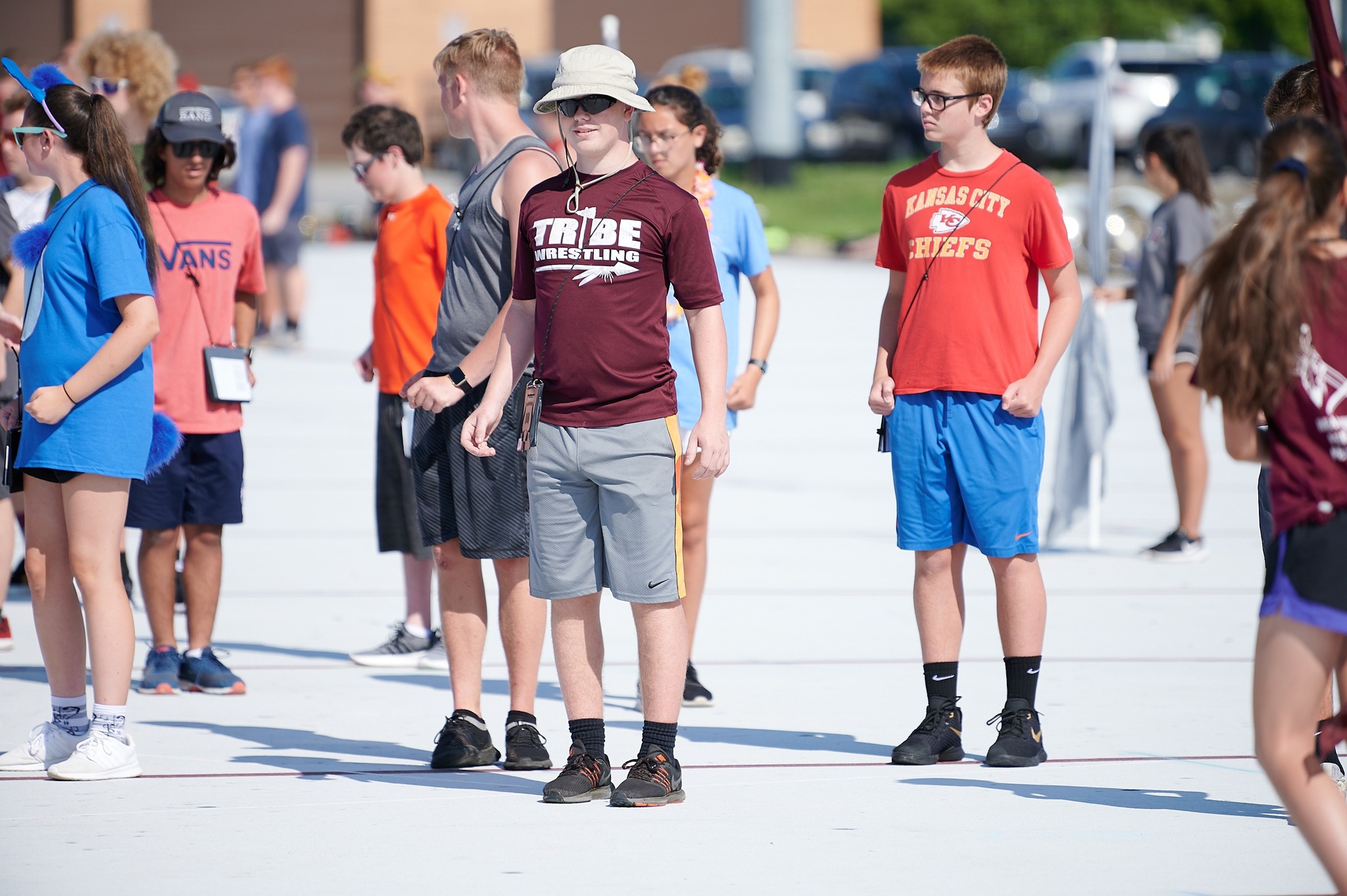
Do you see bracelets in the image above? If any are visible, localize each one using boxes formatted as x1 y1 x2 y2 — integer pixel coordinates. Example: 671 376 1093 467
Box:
448 366 472 394
748 359 767 373
63 383 77 404
236 346 254 364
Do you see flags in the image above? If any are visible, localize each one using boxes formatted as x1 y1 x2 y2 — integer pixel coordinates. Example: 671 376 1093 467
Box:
1043 38 1120 544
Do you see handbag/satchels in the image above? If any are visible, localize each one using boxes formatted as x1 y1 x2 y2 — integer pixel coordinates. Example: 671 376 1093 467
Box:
515 379 545 455
204 344 256 403
876 414 891 454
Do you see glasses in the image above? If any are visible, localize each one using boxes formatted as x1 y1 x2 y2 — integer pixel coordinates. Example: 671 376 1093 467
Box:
910 86 985 112
557 94 618 116
631 121 699 154
12 126 68 148
351 151 382 179
88 75 129 98
171 142 220 158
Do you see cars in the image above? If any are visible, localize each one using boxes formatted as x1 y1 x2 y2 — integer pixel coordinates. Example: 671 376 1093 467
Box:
1134 50 1318 184
821 50 1048 175
1039 37 1216 169
450 59 576 172
648 50 878 169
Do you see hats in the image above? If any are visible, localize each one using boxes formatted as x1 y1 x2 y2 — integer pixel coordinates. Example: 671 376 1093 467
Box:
533 44 656 118
157 89 225 143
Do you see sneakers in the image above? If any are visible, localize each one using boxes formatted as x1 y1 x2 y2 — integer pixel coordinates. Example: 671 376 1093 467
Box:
890 696 966 766
429 713 501 768
136 643 184 695
504 717 554 769
543 739 615 804
682 665 713 706
983 698 1047 768
610 745 687 807
46 729 143 781
0 719 92 772
180 647 246 695
350 621 448 669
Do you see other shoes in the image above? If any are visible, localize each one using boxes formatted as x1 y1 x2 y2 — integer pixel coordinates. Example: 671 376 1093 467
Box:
1136 532 1204 562
0 616 14 649
1320 755 1347 799
10 559 28 588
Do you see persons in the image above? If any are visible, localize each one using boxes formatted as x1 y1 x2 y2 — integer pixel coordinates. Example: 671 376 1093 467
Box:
868 36 1083 767
230 54 311 345
123 91 267 696
0 28 185 652
461 45 729 808
635 85 779 714
0 57 161 783
1175 59 1347 896
341 104 455 668
1093 123 1219 560
398 27 564 770
